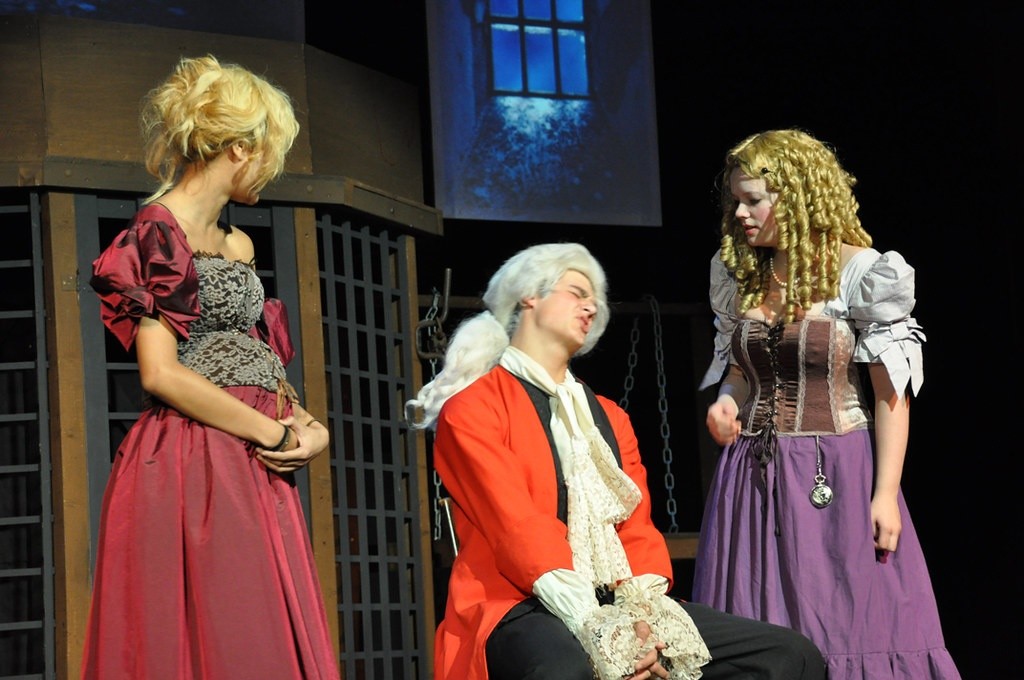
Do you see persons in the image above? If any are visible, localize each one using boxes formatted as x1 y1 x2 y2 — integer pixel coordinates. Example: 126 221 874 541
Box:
81 52 341 680
402 242 827 680
692 129 962 680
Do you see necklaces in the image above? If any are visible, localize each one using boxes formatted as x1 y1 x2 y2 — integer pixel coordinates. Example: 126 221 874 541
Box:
770 256 800 288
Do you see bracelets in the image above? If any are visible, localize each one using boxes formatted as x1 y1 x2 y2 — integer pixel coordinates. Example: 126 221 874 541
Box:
269 423 290 452
306 419 322 426
